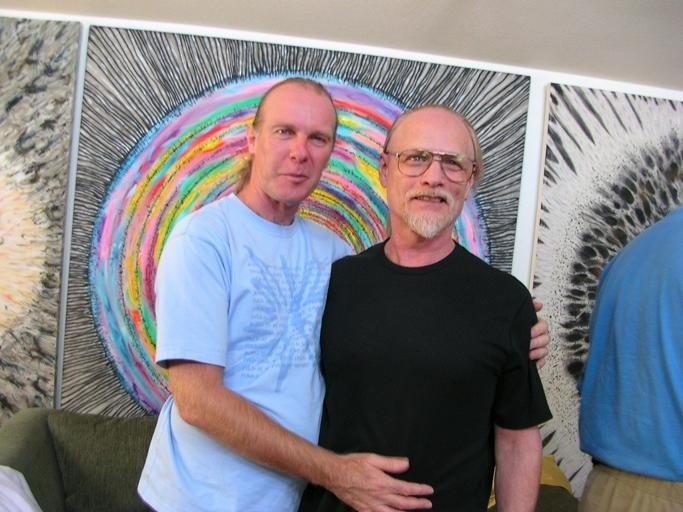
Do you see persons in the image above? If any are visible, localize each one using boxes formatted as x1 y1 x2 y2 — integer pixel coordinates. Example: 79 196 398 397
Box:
579 205 683 512
137 77 551 512
299 104 554 510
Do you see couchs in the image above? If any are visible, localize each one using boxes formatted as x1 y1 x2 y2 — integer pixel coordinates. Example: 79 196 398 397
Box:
0 403 581 512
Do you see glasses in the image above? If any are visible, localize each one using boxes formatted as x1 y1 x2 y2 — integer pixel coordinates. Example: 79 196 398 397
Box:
382 147 476 186
